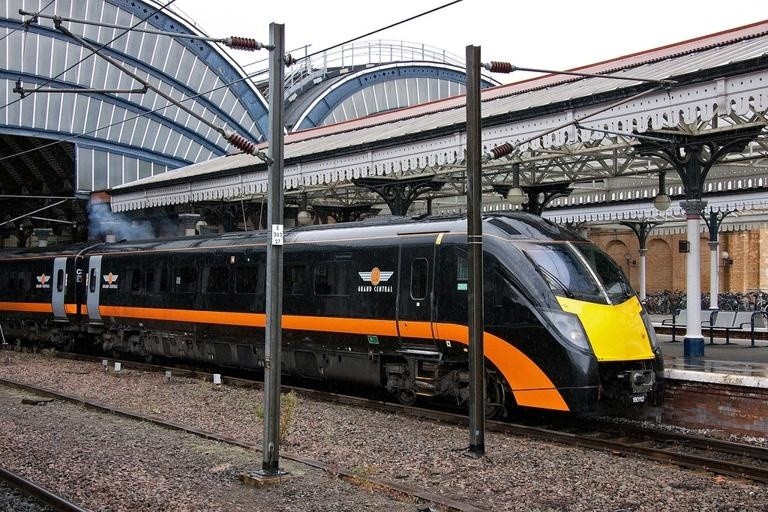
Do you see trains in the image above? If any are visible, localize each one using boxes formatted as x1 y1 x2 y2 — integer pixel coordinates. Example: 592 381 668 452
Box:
0 210 665 420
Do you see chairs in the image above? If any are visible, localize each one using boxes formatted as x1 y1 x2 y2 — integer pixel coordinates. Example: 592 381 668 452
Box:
653 309 768 348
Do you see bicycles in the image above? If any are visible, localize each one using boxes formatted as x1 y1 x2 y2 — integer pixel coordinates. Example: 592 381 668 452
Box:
641 288 768 319
